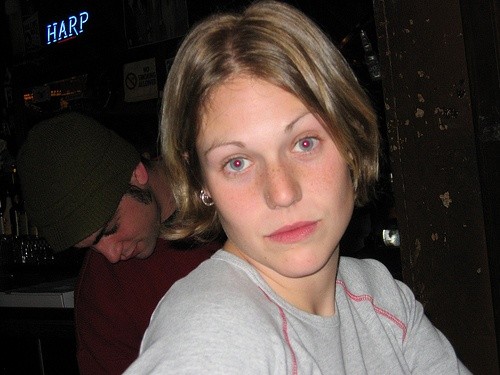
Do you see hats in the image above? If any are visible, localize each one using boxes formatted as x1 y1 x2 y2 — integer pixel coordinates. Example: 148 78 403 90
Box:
18 115 141 251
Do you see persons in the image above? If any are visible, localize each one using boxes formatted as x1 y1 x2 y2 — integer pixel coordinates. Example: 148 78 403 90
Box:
123 2 472 375
19 113 226 375
